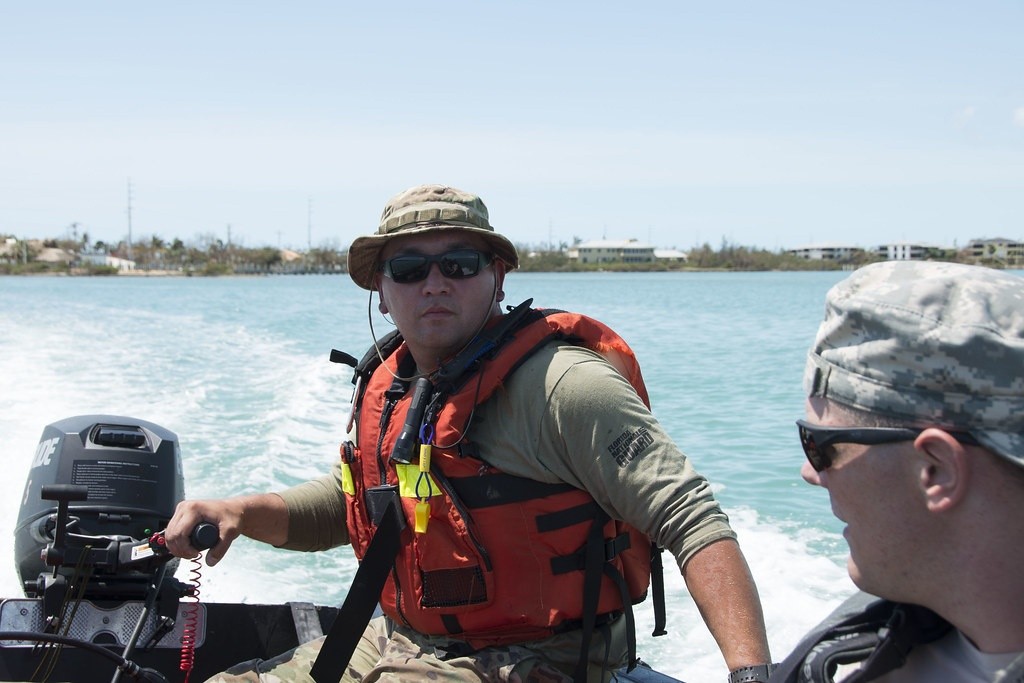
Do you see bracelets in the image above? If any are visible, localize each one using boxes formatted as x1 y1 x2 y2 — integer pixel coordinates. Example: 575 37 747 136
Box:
728 662 781 683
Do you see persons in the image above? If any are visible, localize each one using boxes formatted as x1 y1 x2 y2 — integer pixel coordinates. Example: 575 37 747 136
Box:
761 259 1024 683
165 183 781 683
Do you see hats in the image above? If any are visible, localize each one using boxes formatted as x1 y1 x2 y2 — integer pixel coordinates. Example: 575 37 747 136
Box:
803 260 1024 465
347 185 519 289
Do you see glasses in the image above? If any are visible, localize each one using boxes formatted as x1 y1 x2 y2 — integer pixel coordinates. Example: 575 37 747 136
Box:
797 418 982 472
372 247 498 285
